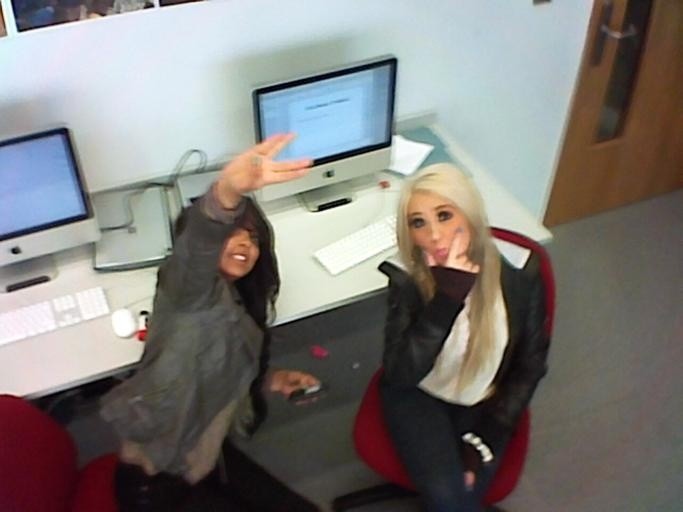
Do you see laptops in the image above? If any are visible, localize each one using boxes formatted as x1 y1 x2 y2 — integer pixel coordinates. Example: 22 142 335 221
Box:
177 170 225 211
94 186 175 271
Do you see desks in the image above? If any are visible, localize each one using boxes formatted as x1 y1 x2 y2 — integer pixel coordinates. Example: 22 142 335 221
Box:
0 124 554 402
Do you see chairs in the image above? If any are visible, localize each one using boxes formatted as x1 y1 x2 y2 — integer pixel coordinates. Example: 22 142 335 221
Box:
350 226 555 502
0 394 118 512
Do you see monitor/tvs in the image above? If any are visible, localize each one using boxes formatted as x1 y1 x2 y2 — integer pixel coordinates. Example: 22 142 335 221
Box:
0 127 101 294
251 54 397 212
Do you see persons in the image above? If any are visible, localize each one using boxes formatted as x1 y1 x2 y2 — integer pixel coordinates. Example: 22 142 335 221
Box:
378 162 548 512
100 131 323 512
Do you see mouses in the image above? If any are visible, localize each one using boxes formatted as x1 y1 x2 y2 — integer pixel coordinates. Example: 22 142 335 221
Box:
112 309 136 337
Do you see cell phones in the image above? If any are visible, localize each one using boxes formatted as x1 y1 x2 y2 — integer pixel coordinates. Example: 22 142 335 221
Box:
287 382 329 400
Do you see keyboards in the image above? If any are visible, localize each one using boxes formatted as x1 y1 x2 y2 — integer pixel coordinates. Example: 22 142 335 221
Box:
312 215 398 276
0 286 110 345
